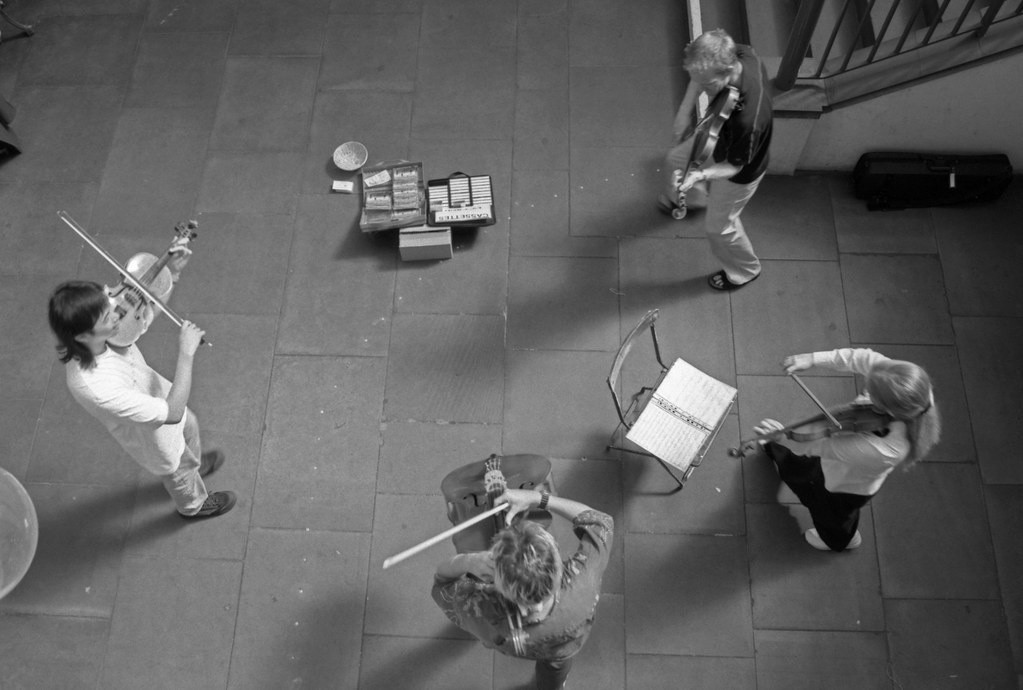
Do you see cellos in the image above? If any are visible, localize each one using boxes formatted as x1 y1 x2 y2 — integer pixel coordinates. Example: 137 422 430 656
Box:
440 452 558 658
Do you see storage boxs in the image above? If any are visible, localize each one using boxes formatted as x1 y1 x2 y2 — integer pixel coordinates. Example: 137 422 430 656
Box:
356 158 495 262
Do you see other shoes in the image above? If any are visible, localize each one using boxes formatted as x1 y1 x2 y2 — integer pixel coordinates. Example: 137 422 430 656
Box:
805 527 862 550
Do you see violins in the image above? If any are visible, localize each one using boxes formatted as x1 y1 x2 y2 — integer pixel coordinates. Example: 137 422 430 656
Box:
102 218 199 349
727 403 890 460
671 82 740 220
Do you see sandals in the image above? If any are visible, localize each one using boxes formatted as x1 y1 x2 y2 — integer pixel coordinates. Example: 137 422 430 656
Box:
708 270 761 291
658 198 677 214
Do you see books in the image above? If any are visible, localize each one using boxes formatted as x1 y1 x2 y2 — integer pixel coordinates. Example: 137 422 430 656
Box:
625 358 738 472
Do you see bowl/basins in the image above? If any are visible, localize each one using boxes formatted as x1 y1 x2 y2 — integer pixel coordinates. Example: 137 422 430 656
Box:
333 141 368 171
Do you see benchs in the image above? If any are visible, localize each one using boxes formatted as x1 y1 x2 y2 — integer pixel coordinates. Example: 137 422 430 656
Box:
605 307 738 491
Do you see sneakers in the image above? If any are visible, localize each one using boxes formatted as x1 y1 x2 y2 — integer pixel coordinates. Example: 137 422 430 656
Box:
177 491 237 518
199 451 225 477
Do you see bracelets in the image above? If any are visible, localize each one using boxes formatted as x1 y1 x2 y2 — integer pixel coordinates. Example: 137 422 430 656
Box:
538 489 548 508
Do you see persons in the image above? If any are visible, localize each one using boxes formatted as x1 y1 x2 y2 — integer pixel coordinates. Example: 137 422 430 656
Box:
659 29 772 290
753 348 940 553
48 237 237 519
430 489 614 690
